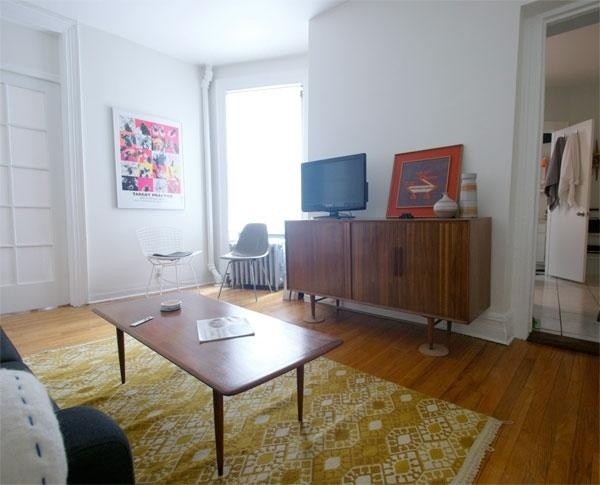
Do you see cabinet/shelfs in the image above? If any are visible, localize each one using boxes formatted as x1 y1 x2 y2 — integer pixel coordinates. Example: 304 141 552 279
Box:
286 221 493 350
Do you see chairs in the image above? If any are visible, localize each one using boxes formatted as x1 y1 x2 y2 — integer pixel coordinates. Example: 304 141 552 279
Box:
136 224 205 296
218 222 272 301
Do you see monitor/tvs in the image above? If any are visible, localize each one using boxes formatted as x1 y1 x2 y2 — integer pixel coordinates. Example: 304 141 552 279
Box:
300 152 368 219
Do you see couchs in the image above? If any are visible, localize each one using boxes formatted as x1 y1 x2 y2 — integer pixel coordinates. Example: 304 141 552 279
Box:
0 323 137 483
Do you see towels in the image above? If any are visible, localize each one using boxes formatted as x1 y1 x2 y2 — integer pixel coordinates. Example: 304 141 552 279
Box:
543 135 583 213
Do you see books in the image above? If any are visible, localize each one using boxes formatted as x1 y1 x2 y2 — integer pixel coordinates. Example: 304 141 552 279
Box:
196 313 256 343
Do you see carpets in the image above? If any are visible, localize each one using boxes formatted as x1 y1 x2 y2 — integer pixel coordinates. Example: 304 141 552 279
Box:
18 331 503 485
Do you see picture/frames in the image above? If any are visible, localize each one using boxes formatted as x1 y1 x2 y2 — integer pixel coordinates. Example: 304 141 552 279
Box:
387 142 465 219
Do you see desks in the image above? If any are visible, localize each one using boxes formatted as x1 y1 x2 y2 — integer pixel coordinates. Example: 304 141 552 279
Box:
91 291 344 478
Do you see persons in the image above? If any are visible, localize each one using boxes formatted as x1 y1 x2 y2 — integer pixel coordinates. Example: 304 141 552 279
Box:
210 318 222 329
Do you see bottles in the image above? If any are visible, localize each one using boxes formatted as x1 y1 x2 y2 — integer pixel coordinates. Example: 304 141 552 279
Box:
432 172 479 218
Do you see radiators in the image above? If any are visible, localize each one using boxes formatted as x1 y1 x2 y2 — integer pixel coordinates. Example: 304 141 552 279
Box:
228 242 284 293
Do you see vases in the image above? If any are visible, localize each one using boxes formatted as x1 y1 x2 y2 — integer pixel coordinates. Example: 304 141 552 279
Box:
433 172 478 218
